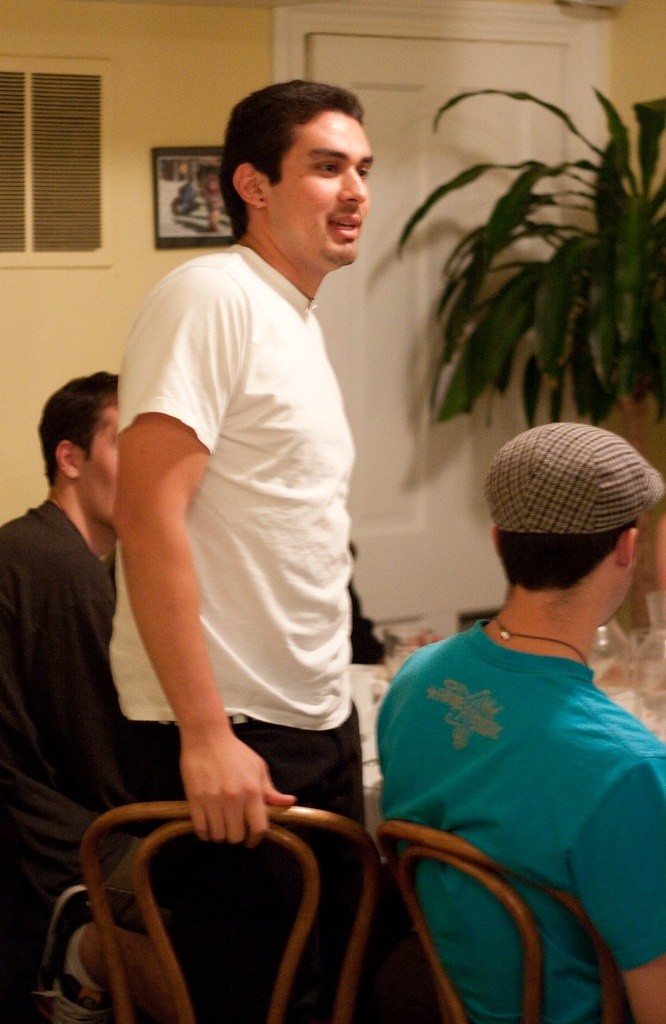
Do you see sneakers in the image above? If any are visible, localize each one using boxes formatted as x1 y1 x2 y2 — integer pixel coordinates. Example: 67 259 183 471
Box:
34 884 105 1024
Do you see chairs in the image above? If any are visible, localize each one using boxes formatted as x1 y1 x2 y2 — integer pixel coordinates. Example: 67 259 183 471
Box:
79 800 629 1024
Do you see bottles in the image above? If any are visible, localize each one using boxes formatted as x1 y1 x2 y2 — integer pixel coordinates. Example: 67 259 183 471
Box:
589 590 666 743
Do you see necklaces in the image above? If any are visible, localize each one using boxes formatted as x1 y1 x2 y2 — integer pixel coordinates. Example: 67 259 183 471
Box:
493 615 587 665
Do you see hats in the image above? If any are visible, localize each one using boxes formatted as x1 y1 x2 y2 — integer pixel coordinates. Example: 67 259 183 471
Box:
485 422 665 533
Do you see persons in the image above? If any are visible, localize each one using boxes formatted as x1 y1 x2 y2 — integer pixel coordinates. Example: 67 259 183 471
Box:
108 80 374 1024
375 422 666 1024
0 371 120 1024
201 168 222 230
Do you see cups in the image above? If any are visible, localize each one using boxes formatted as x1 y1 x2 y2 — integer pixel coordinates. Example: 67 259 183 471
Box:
350 664 388 744
